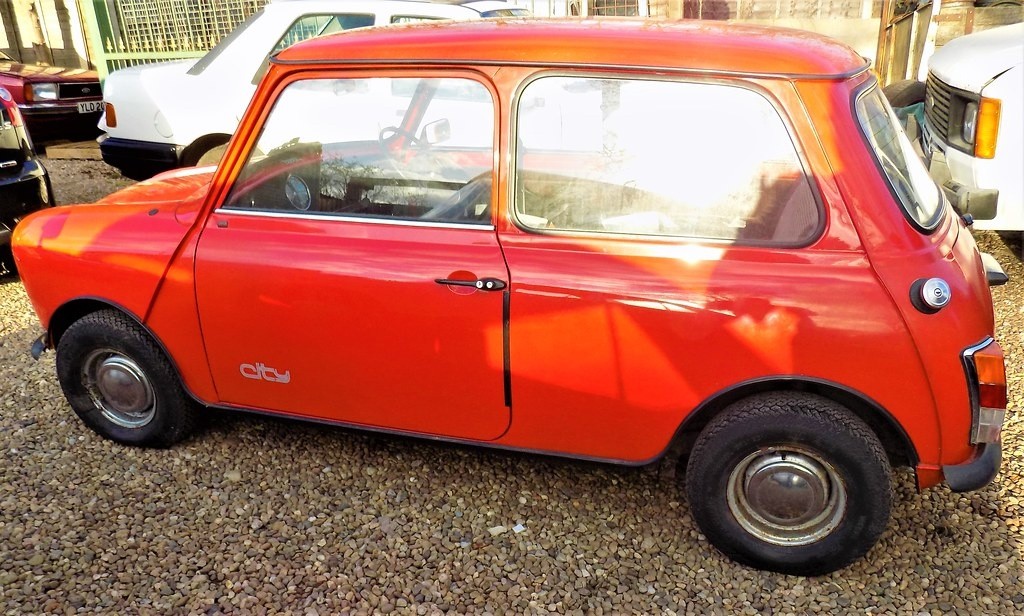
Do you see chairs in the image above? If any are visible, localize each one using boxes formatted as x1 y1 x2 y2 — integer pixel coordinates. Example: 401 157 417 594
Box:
423 174 493 222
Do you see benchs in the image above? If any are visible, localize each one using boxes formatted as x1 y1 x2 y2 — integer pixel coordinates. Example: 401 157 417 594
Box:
738 149 818 238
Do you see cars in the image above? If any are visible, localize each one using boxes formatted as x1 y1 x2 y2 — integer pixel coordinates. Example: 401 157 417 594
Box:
95 0 528 184
0 87 58 254
0 53 105 139
924 20 1024 258
20 21 1010 571
